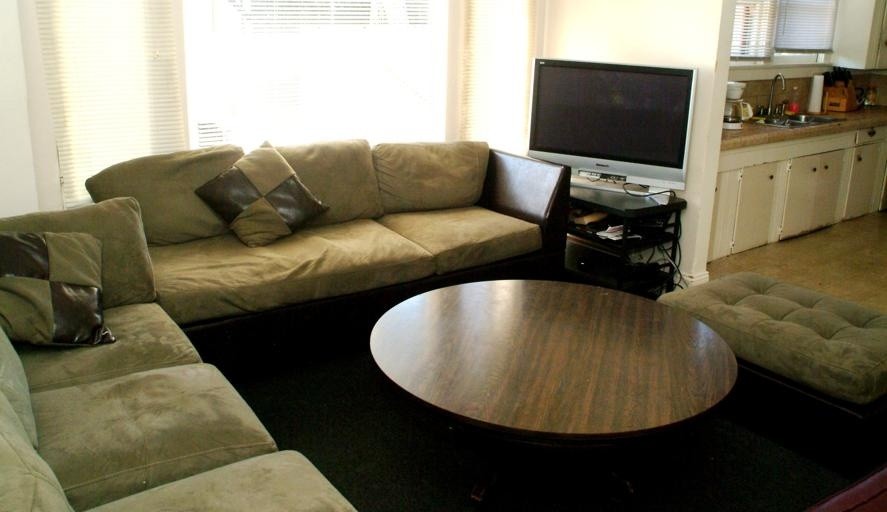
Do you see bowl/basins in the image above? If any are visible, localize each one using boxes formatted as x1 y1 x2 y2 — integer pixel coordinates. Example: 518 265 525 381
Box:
726 82 746 99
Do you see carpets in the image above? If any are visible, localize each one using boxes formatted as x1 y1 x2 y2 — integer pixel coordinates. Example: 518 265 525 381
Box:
169 261 887 512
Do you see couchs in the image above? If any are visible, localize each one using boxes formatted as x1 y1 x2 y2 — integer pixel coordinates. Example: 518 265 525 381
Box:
85 138 435 337
0 301 204 391
377 149 571 284
0 362 275 509
80 449 353 512
655 270 887 404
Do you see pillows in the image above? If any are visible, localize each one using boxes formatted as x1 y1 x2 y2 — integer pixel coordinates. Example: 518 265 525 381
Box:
259 142 385 228
87 146 244 245
372 144 488 215
0 231 117 349
193 139 329 247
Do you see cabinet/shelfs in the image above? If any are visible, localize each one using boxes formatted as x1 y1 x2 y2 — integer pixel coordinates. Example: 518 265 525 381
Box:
833 0 887 70
704 138 786 262
845 123 887 221
779 133 855 244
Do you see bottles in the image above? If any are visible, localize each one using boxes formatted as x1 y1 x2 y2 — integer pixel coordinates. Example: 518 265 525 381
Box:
790 86 801 114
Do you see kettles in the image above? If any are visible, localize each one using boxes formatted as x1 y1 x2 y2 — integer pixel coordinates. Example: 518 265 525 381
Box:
723 99 753 130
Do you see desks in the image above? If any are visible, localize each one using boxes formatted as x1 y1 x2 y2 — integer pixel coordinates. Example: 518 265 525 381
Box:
561 187 687 297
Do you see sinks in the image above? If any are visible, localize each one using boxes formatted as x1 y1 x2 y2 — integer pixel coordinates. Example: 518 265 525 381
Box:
754 114 847 129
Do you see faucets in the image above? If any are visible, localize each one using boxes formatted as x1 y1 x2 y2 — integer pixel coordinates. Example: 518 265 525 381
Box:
758 73 787 117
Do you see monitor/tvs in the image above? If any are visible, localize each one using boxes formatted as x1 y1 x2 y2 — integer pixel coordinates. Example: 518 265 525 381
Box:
527 57 699 196
